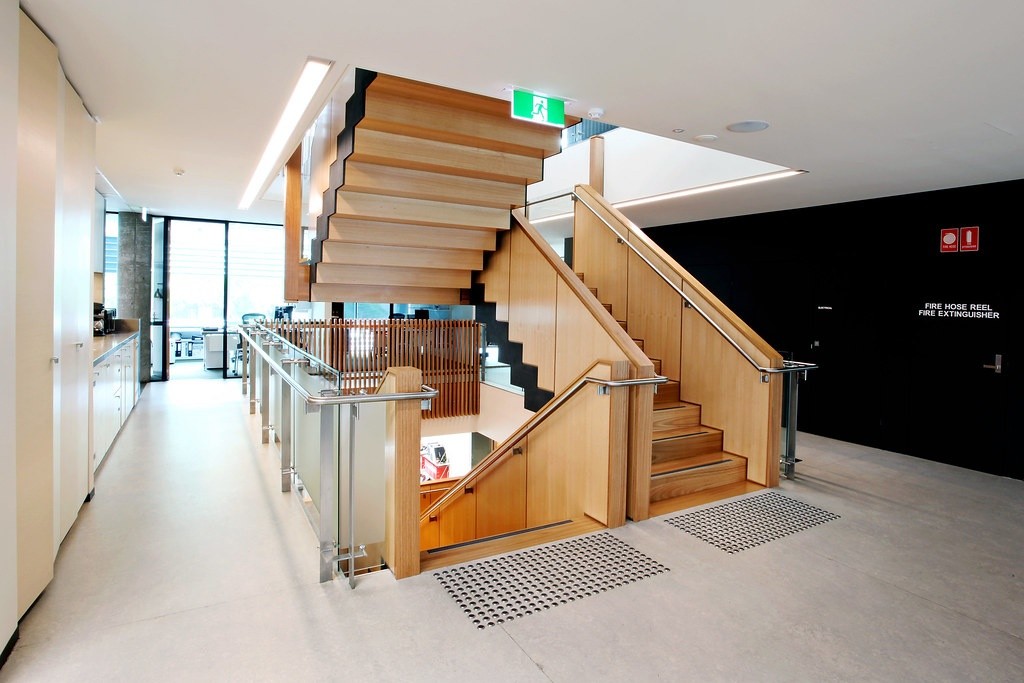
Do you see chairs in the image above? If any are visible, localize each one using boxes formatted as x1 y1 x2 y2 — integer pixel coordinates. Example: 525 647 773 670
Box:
387 312 405 329
235 312 267 374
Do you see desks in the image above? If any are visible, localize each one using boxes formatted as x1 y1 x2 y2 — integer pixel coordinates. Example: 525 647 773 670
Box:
372 326 446 358
200 331 312 369
